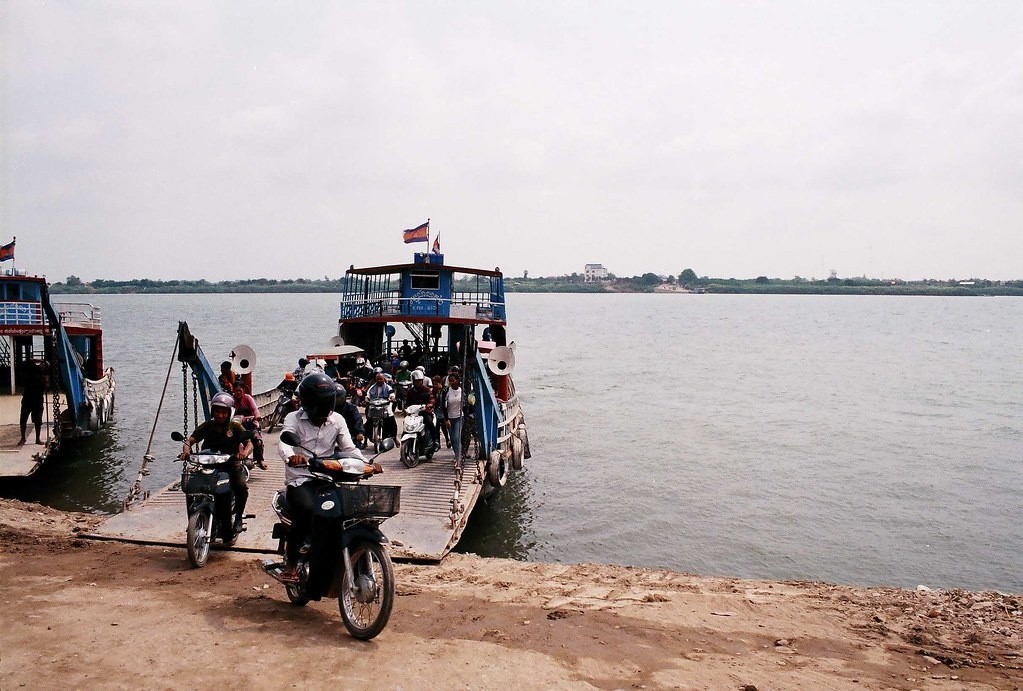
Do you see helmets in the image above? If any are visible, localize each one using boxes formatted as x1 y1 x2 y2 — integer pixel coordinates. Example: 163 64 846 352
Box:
211 392 235 408
410 370 424 380
356 358 365 368
373 367 383 374
400 361 409 369
416 366 425 374
299 373 336 417
335 384 346 408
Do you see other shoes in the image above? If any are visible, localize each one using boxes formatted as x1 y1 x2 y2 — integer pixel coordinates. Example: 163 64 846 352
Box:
447 441 452 449
18 439 26 445
463 452 471 459
233 515 242 533
395 439 400 448
361 444 368 450
433 440 439 452
36 440 44 445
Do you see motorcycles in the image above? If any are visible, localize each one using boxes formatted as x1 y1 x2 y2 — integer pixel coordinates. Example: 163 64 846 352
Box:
231 414 258 471
266 385 299 434
261 431 402 640
345 348 440 411
359 389 395 453
170 429 257 568
397 393 440 468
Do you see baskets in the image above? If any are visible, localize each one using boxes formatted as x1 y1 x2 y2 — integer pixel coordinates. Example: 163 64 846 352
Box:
181 472 220 493
339 484 401 517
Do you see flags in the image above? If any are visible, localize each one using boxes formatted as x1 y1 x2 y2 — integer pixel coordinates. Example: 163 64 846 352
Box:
0 241 15 262
402 222 429 244
432 236 440 255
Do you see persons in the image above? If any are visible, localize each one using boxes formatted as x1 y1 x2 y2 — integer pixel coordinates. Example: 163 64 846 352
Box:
277 374 383 579
232 380 269 471
277 337 482 470
16 359 45 446
180 391 249 534
218 361 237 395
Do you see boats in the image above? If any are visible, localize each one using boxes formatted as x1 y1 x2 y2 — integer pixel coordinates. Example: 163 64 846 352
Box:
653 282 693 293
338 253 528 504
0 267 116 441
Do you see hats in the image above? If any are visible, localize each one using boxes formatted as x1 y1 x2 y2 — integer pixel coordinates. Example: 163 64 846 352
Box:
284 373 295 381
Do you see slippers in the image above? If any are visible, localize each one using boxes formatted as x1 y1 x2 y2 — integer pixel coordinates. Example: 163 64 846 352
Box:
256 461 268 470
280 571 299 582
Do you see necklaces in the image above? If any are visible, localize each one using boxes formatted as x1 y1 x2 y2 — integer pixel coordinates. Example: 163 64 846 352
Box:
452 389 458 397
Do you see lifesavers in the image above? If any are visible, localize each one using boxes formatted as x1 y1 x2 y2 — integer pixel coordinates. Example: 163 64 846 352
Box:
518 429 532 459
488 449 510 487
510 438 526 472
87 394 116 433
